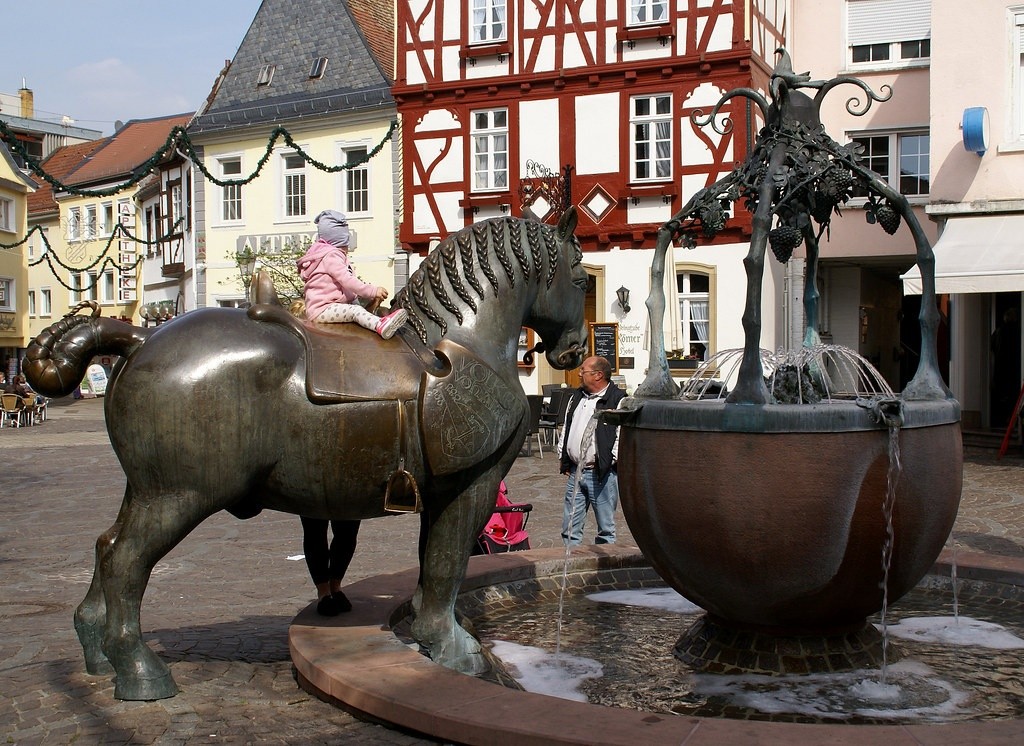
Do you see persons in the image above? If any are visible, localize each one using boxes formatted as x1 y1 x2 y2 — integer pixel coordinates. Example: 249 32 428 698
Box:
556 355 629 547
0 370 49 428
296 209 408 339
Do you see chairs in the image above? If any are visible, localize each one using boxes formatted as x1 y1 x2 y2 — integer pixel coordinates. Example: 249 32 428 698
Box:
0 390 53 429
522 384 577 458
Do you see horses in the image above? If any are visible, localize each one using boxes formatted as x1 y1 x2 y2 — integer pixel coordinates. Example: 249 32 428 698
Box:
19 199 589 705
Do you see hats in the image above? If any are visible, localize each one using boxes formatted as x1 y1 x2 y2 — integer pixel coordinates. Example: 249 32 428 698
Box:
314 210 349 247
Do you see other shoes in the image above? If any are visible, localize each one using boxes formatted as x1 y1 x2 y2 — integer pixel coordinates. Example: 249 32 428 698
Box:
377 308 408 340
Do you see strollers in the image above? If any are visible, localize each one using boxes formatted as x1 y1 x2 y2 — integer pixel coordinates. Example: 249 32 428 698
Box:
468 482 533 556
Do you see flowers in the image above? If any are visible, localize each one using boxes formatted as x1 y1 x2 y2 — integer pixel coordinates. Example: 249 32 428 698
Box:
665 348 698 360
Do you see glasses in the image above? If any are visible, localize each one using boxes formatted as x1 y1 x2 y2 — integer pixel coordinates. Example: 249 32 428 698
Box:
581 368 601 378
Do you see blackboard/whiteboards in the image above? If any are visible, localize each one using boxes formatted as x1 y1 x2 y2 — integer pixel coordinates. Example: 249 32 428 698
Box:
589 321 619 376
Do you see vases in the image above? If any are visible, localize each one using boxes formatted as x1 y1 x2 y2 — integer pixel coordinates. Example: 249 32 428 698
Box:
667 359 700 369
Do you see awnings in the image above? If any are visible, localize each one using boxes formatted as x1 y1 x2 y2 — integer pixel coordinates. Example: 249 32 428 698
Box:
899 214 1024 296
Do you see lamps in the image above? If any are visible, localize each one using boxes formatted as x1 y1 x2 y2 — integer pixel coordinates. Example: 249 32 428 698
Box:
616 285 631 314
